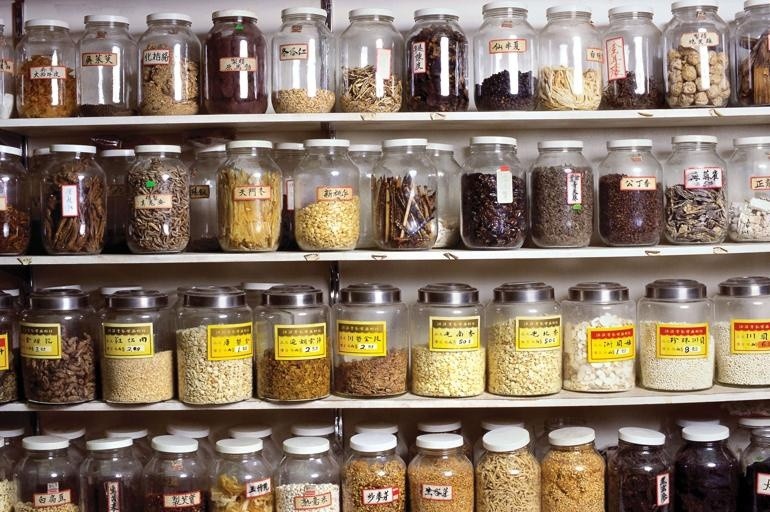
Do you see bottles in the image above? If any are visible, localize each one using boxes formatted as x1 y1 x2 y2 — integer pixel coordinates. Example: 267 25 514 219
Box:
144 11 204 115
473 1 538 110
663 0 729 109
486 278 564 397
716 275 769 389
338 8 406 113
18 19 75 118
407 7 470 114
176 288 256 407
256 285 334 405
241 281 284 308
24 284 103 411
729 0 770 107
411 280 487 402
0 418 770 511
208 9 269 114
0 19 21 120
80 15 139 117
272 6 337 114
564 276 636 393
103 289 176 408
538 4 605 110
2 288 24 409
2 133 769 254
334 282 412 400
604 4 663 109
638 278 715 392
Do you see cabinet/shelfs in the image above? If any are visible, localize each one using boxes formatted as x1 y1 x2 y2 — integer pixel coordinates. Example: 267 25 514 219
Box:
0 101 770 416
